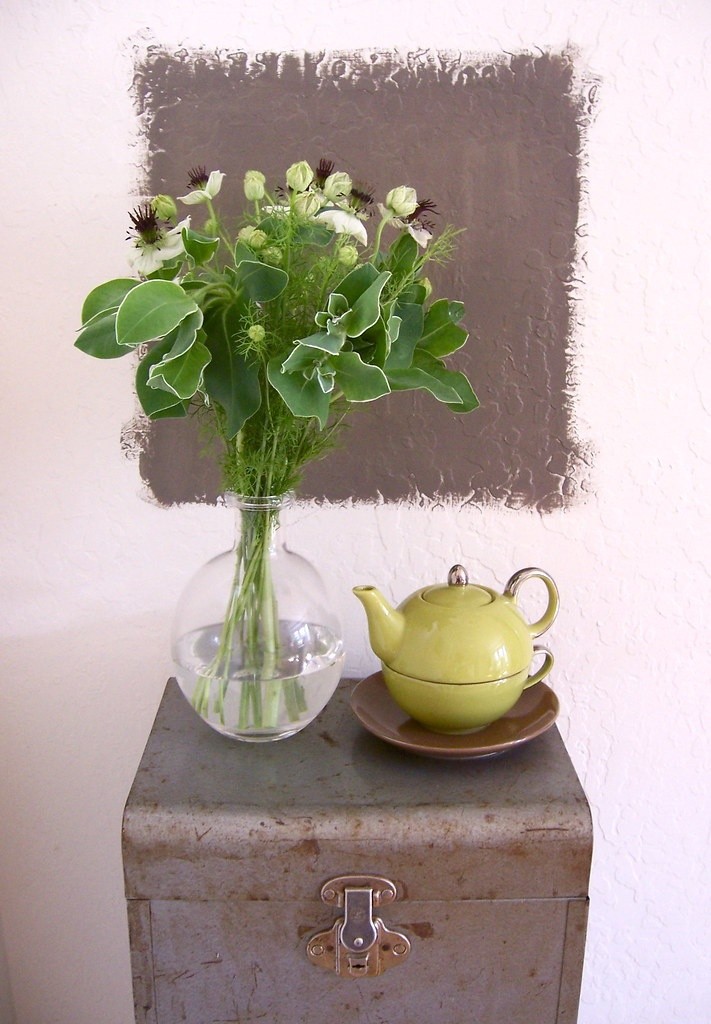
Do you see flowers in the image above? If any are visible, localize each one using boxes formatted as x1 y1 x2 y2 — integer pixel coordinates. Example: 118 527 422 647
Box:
73 160 483 731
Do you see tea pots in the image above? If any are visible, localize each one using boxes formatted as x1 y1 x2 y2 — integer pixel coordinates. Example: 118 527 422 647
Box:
351 564 560 736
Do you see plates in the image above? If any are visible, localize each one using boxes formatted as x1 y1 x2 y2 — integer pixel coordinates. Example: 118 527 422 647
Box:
351 668 560 760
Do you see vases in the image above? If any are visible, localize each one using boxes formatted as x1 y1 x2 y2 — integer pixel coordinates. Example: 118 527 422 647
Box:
170 485 345 744
